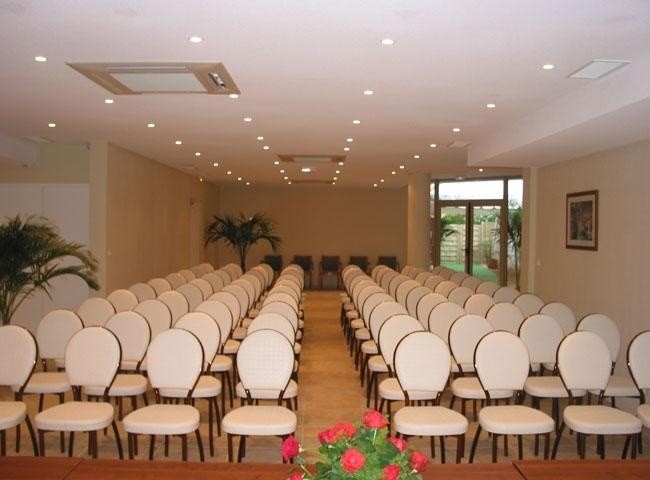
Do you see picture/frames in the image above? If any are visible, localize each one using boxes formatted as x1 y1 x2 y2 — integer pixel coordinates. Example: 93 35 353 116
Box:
565 189 599 251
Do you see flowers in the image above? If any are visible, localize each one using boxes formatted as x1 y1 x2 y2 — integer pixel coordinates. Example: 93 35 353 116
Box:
282 408 432 480
